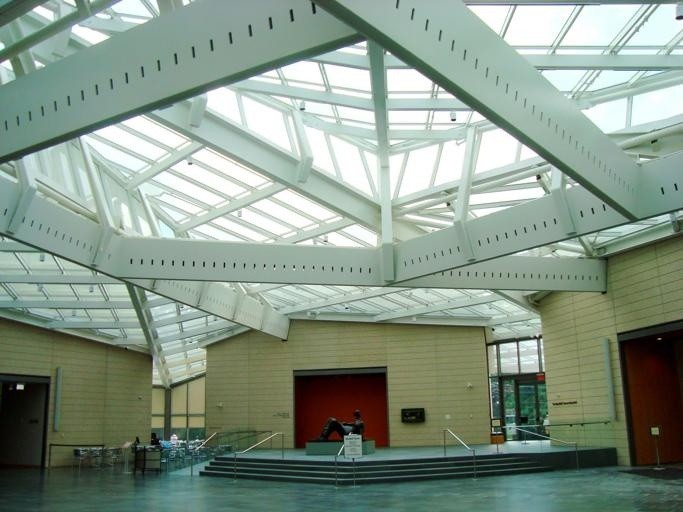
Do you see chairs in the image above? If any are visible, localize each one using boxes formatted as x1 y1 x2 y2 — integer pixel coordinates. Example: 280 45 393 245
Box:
70 438 221 477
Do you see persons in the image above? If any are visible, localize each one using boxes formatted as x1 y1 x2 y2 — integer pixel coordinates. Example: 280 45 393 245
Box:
316 410 364 442
151 432 163 452
